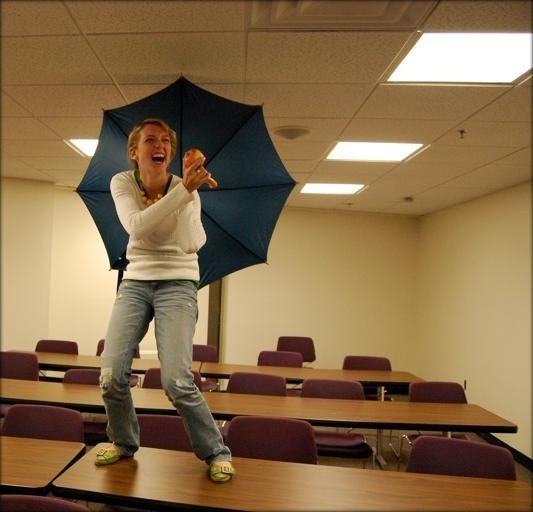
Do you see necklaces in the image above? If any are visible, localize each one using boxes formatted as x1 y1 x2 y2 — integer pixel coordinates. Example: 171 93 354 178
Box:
135 170 173 206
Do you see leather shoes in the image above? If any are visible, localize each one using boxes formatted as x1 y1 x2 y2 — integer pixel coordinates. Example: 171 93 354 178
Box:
94 448 124 466
209 459 233 482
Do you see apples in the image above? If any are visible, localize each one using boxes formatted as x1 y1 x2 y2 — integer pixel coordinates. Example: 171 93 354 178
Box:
183 150 204 171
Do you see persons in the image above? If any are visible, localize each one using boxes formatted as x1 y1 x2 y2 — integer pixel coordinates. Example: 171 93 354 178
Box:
95 118 235 483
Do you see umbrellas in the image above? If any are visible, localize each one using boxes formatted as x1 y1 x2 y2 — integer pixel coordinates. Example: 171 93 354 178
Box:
72 72 300 289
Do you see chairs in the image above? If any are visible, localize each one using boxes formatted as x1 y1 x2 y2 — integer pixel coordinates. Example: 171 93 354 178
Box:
0 336 533 512
